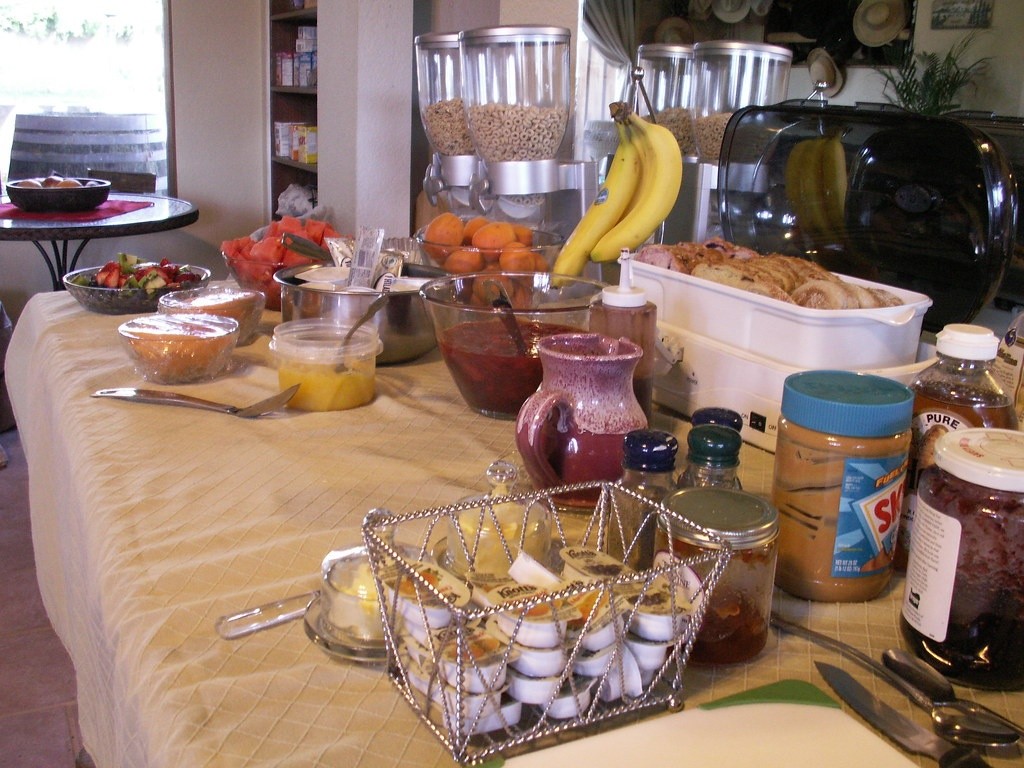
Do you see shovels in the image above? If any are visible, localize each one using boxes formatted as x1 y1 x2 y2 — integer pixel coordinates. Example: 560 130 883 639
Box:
89 384 304 416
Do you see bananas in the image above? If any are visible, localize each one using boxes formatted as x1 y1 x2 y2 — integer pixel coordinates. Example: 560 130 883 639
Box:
548 102 682 289
784 126 850 244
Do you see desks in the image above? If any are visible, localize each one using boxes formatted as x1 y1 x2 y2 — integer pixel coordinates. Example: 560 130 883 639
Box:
3 282 1024 768
0 193 198 291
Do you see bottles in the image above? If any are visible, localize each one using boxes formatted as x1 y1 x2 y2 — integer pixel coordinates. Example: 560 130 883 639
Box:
677 423 742 490
588 259 657 419
993 310 1024 425
892 322 1018 575
693 408 743 433
607 428 679 568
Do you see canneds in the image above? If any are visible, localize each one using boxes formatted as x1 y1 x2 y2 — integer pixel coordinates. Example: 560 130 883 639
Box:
898 429 1024 693
768 367 912 603
652 485 777 669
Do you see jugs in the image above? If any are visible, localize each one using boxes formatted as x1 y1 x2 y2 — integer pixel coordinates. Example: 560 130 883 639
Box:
516 333 648 508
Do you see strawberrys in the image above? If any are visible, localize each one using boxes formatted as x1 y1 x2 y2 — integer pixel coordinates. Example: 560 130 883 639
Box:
95 257 202 292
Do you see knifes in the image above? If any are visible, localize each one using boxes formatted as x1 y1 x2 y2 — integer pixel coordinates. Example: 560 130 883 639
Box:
814 662 990 768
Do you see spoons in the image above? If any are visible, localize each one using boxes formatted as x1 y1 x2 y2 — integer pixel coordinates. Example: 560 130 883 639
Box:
90 382 301 417
768 612 1020 748
881 646 1024 737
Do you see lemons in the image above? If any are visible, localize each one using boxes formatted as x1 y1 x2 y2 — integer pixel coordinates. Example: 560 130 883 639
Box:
72 253 193 308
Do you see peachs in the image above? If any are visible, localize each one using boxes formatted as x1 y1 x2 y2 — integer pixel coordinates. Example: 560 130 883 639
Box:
424 212 549 310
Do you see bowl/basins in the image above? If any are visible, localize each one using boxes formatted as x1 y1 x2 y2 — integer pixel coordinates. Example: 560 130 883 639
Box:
266 316 384 411
221 249 313 312
62 263 212 316
157 286 266 347
419 272 612 420
414 223 565 275
272 262 450 364
5 178 111 211
118 311 239 382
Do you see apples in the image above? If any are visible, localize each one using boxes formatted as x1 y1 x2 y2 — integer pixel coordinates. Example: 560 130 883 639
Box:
15 175 85 187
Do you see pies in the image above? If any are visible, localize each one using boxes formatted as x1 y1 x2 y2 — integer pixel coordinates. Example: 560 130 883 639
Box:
634 238 903 309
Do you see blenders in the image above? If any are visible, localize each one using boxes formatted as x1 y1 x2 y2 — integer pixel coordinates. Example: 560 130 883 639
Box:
414 22 792 244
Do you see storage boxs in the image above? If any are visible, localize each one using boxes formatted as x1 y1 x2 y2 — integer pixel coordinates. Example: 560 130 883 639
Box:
274 0 317 164
609 249 948 453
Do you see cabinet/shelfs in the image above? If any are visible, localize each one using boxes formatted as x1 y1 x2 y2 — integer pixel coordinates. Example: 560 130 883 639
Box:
271 6 319 174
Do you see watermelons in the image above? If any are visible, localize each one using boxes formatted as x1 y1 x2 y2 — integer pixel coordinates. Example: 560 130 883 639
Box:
221 216 352 310
